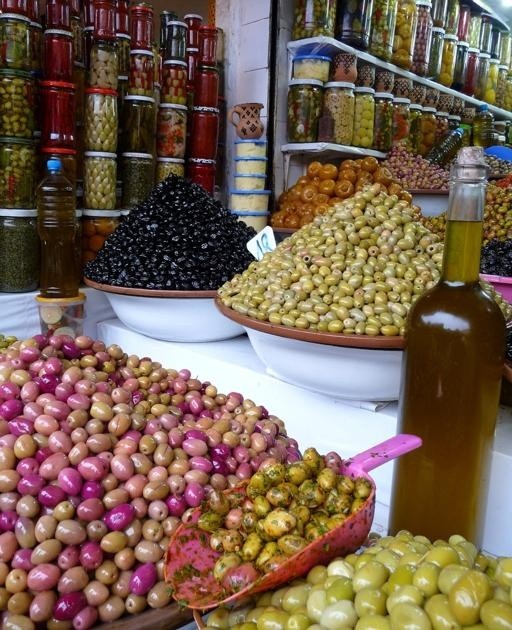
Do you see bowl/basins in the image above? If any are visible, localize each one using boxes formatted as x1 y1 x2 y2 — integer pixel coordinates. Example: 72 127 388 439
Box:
215 291 512 403
270 226 299 246
479 272 512 305
403 187 450 219
82 277 247 344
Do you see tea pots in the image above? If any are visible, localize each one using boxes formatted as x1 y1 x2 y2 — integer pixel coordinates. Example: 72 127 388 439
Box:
227 102 264 139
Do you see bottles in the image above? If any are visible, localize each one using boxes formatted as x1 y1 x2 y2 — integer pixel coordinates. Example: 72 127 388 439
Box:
0 0 223 299
273 0 512 174
389 145 507 555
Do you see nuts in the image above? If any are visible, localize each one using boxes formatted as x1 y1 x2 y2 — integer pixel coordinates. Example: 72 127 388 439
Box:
478 236 512 277
84 173 258 289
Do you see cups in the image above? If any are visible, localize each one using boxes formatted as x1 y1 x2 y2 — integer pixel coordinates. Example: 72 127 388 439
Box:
34 291 87 342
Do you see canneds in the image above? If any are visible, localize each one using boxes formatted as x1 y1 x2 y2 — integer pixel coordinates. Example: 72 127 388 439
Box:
287 0 511 179
1 0 228 290
